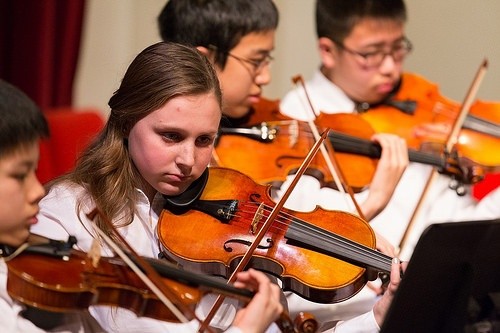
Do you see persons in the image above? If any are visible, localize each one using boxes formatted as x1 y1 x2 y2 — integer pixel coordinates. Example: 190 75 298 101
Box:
28 41 410 333
158 0 410 295
280 0 500 262
0 77 284 333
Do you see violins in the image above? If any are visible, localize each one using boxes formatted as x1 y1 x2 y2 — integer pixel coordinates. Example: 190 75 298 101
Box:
0 236 319 333
352 73 500 171
155 167 404 305
209 95 485 195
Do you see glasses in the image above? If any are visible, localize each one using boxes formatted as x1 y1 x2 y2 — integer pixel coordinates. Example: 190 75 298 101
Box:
206 43 277 72
330 34 413 63
